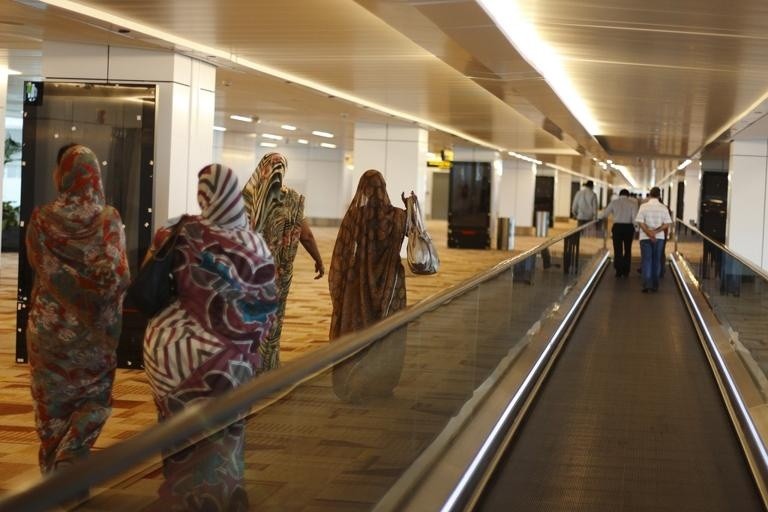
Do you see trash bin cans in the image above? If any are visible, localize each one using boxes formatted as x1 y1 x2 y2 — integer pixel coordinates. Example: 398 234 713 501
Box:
536 211 550 237
498 217 515 250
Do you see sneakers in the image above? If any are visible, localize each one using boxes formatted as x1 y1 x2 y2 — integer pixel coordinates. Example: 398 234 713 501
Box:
642 288 651 293
614 273 622 278
623 273 630 277
652 288 659 292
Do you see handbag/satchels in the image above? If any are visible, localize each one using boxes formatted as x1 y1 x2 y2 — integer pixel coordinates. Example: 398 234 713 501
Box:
407 228 440 274
128 256 173 317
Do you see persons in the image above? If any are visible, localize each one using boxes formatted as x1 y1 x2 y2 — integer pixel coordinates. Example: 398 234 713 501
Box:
637 198 670 277
598 188 639 280
242 151 325 376
635 187 672 293
25 143 131 479
630 193 650 239
572 180 598 227
328 169 418 403
139 164 279 512
